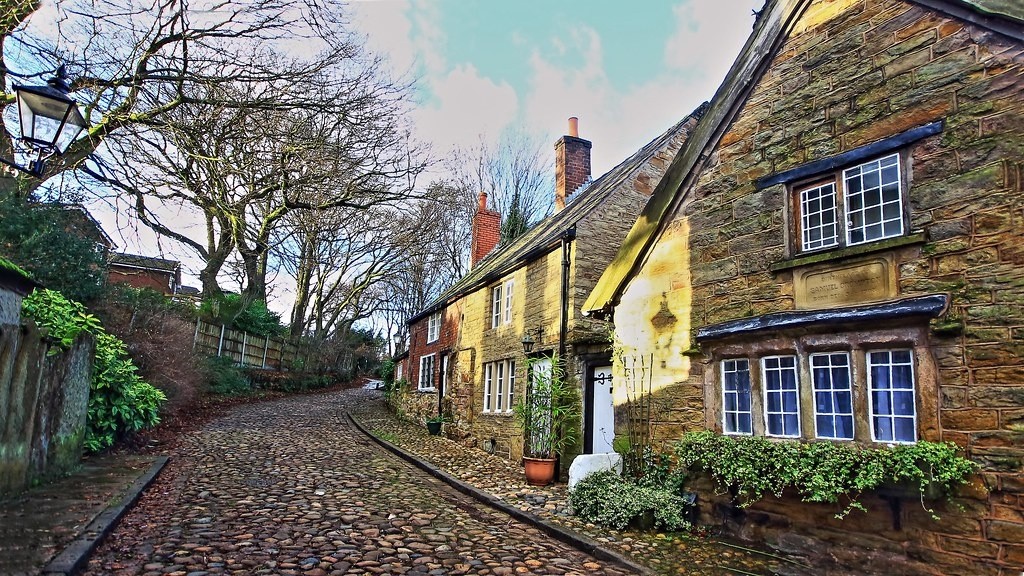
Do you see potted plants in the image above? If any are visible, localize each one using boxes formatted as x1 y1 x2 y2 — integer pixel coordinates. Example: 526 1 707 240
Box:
513 350 578 485
570 471 687 532
427 415 444 435
673 431 968 518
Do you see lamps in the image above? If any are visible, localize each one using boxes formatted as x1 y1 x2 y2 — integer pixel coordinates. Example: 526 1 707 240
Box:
520 326 544 356
0 63 89 179
